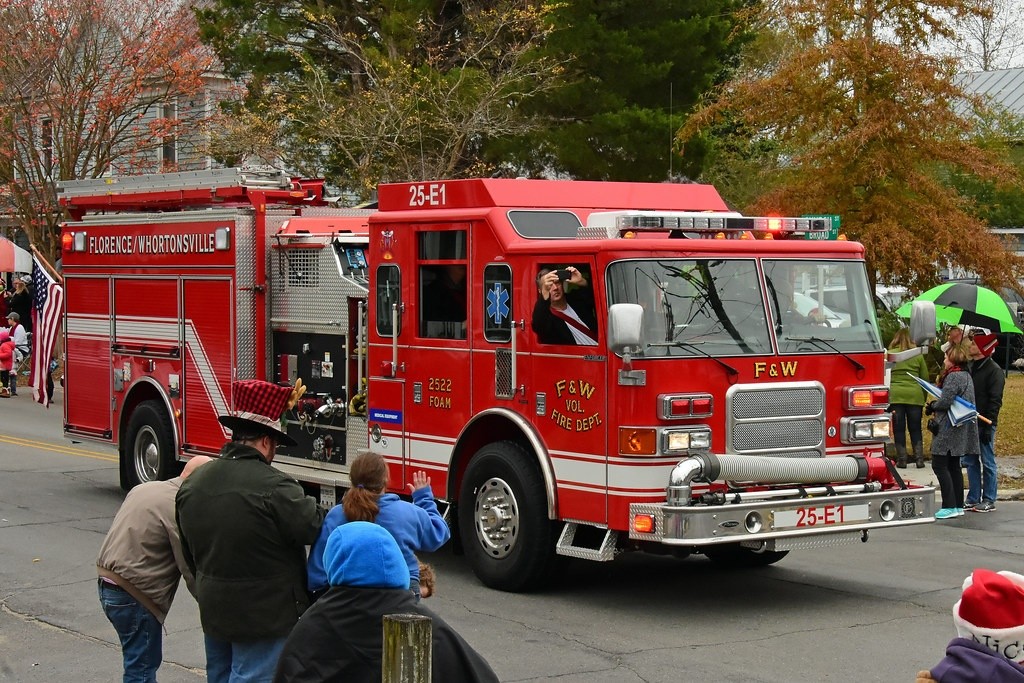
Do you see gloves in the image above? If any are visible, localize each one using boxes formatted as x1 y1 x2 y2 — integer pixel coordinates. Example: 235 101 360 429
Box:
925 399 937 416
979 425 991 446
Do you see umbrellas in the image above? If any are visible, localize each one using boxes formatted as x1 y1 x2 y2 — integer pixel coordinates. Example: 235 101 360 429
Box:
0 235 33 274
895 283 1024 346
906 372 992 428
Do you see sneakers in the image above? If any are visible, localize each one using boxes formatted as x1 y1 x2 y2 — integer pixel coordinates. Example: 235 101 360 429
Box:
973 500 996 513
962 500 982 510
957 507 965 516
935 507 958 519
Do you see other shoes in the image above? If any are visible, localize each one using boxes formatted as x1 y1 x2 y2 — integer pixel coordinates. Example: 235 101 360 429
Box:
49 399 54 403
11 394 18 397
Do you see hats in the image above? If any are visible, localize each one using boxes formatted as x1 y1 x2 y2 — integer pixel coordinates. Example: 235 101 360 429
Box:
5 312 20 320
973 333 998 357
217 379 300 447
952 568 1024 664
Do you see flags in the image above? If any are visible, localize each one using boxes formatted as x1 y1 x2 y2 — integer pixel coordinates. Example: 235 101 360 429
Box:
28 255 64 409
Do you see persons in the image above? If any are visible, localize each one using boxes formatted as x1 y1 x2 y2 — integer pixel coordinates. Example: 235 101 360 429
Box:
96 455 212 683
0 275 33 397
55 246 62 275
174 378 330 683
272 521 499 683
913 568 1024 683
756 280 825 324
48 370 54 404
941 325 973 361
307 452 450 599
418 563 434 599
925 345 981 518
888 329 930 468
531 266 599 345
964 334 1005 511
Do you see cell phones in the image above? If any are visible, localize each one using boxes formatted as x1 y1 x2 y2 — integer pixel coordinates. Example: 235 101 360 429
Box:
555 270 571 279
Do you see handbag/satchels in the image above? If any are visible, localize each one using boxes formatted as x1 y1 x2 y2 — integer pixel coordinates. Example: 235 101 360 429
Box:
927 418 939 437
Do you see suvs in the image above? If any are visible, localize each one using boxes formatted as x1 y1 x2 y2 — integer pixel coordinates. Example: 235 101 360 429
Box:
762 255 1024 329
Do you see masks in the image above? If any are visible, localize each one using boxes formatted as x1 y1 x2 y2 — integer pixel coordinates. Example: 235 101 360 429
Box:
0 286 4 291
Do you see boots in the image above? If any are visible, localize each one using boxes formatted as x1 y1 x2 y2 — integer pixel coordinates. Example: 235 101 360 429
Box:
894 442 907 469
0 386 11 398
912 441 925 468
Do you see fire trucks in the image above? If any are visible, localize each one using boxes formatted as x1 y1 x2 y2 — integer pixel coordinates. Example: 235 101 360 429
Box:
55 167 937 592
